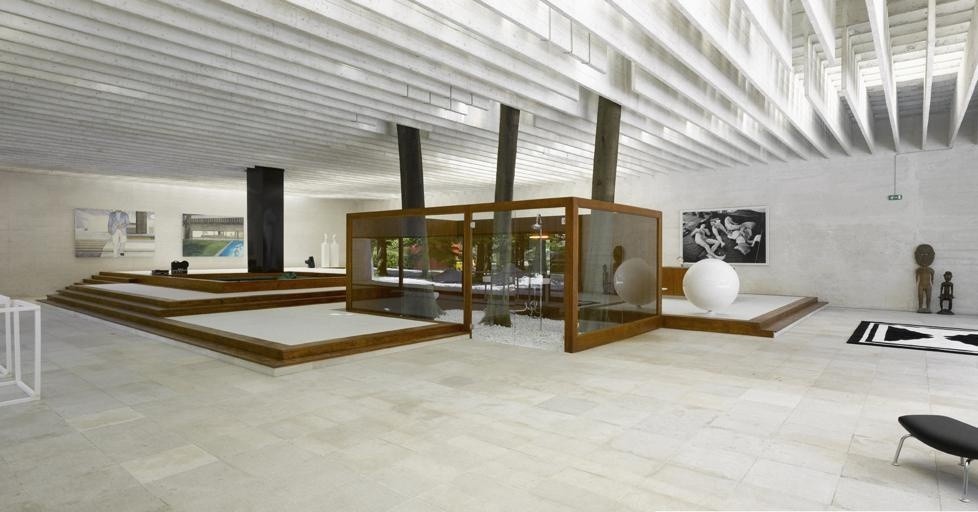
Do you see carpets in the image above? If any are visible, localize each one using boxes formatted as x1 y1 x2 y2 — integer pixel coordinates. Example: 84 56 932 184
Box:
846 320 978 356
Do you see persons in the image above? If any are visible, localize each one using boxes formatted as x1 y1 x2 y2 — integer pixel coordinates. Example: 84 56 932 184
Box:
108 210 130 258
938 271 955 314
914 244 936 314
689 215 761 261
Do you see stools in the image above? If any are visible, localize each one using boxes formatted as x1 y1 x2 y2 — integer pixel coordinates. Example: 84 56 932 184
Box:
890 413 978 503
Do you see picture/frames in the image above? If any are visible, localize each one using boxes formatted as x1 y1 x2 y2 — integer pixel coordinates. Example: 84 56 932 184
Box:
679 207 770 265
